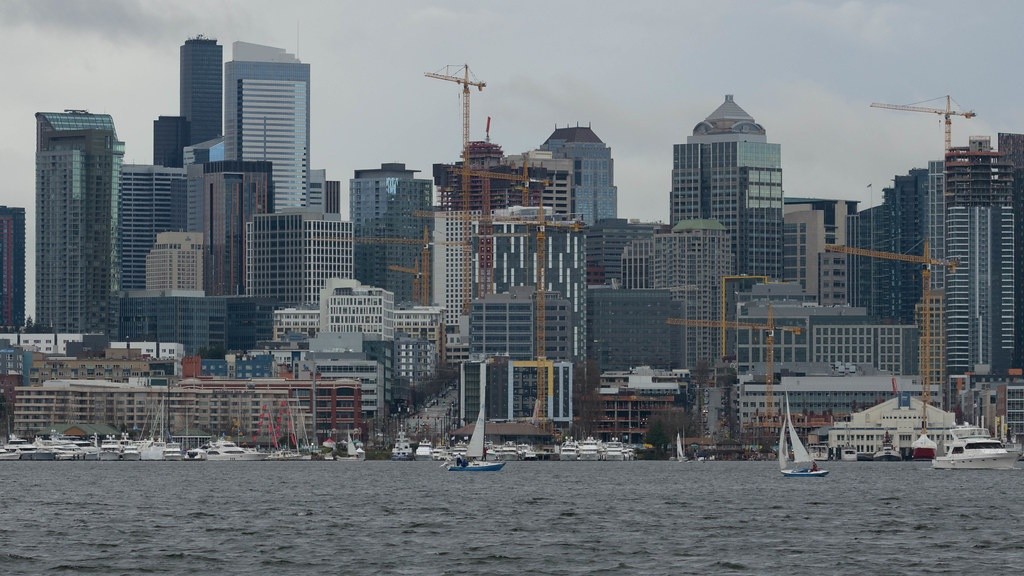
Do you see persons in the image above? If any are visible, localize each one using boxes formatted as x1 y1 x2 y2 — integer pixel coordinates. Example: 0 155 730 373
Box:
810 461 819 473
456 445 488 466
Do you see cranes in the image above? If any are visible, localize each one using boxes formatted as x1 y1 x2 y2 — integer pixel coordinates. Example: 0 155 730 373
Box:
312 64 588 431
668 304 802 421
827 240 960 434
871 94 977 153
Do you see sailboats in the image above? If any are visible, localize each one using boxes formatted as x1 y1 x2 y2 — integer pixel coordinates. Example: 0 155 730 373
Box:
840 417 857 460
440 404 506 471
779 390 830 477
676 432 688 462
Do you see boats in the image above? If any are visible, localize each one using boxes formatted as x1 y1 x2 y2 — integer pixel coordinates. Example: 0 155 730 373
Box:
809 445 829 461
932 421 1019 470
0 396 638 462
872 427 901 461
910 434 937 460
